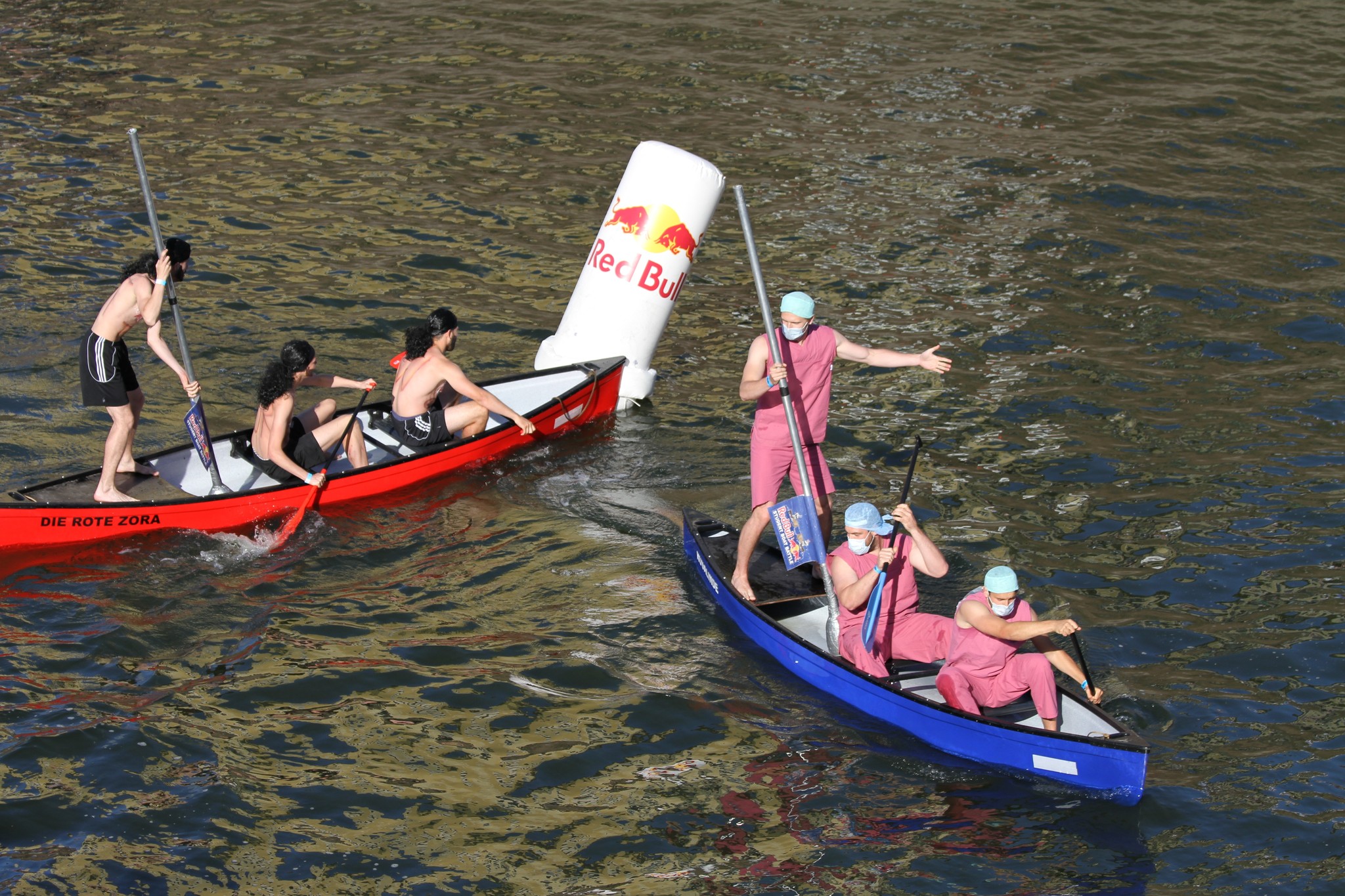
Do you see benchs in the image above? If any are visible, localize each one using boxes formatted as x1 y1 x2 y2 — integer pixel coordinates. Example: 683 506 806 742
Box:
941 697 1036 718
835 656 930 671
372 419 452 453
234 447 317 483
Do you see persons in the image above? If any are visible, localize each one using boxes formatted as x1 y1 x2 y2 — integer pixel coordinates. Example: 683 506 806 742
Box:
934 565 1103 733
390 308 536 446
243 339 378 489
728 291 952 602
823 503 955 680
79 237 201 503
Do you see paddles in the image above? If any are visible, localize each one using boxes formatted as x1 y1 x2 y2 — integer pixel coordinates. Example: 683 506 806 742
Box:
389 351 523 433
1071 632 1101 708
861 434 923 653
277 383 376 546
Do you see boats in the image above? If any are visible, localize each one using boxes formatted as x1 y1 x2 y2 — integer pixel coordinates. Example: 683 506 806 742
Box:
680 505 1150 802
0 356 626 548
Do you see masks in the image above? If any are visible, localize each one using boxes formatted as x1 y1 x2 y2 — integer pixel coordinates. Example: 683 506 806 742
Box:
986 591 1016 616
781 322 809 341
847 530 874 556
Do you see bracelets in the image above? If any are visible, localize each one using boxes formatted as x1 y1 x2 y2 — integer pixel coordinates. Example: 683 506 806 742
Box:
766 375 773 388
155 278 167 286
874 565 882 574
1081 680 1088 691
303 472 312 484
